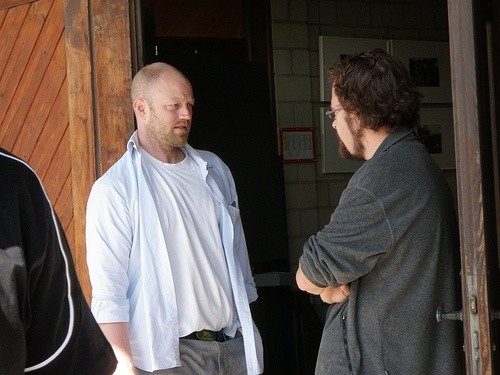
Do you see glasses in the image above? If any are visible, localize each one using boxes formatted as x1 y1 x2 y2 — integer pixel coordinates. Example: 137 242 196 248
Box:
325 106 349 119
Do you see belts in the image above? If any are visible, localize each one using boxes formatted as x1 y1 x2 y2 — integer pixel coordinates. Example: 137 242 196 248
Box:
178 329 241 342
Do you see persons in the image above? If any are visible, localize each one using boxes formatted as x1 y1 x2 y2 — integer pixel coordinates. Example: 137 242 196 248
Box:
86 63 263 375
0 146 118 375
296 49 466 375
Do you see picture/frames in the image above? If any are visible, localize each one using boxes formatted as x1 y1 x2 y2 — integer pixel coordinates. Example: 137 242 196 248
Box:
278 127 318 164
